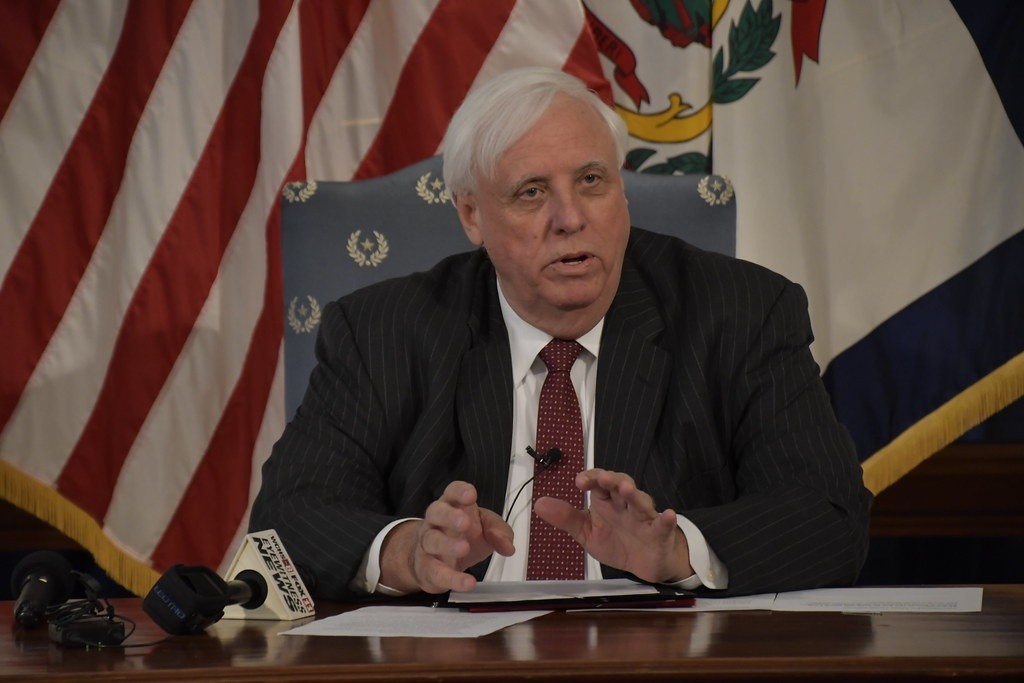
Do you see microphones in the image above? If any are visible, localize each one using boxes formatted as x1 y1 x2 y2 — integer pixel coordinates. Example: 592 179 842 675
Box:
527 444 562 469
141 528 320 635
10 549 76 628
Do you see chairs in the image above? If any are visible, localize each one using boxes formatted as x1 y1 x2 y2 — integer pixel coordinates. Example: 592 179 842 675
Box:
280 153 736 424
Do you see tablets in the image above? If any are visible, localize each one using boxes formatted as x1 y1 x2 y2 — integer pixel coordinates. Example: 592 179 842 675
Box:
465 585 696 612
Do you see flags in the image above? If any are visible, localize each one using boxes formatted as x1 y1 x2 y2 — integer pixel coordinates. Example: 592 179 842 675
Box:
0 0 1024 598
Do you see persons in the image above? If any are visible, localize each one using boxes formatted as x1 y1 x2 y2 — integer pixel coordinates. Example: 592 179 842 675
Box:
249 69 868 594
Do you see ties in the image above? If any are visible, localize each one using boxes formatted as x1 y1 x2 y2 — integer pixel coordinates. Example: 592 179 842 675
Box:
526 339 588 580
